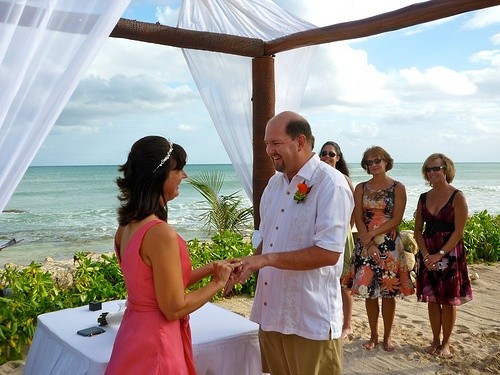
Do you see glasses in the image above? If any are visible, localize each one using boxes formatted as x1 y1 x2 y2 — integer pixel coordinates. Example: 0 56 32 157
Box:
366 159 382 165
321 151 337 157
427 166 445 172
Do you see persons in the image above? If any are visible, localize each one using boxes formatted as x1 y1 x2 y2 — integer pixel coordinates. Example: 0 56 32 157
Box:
225 111 355 375
318 141 356 339
414 153 473 357
104 136 242 374
342 146 415 351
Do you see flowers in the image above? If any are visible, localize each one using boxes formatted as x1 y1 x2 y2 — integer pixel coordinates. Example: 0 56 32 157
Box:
296 182 308 194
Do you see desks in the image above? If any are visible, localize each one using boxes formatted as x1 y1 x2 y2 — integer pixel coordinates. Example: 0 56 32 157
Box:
35 303 260 375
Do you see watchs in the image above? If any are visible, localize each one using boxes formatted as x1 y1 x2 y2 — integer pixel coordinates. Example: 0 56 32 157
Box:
440 250 445 256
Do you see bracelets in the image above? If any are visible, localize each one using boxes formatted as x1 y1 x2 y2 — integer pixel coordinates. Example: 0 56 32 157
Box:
366 244 374 250
425 253 429 257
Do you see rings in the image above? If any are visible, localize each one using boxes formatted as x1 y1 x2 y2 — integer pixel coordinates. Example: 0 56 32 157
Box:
371 254 374 257
374 252 376 255
239 261 243 264
433 264 435 266
425 259 428 262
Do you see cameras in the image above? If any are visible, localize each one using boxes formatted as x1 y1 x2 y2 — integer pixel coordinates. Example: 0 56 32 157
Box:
437 262 443 269
380 255 388 259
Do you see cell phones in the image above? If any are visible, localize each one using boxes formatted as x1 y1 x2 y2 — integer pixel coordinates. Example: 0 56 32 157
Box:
77 326 105 337
